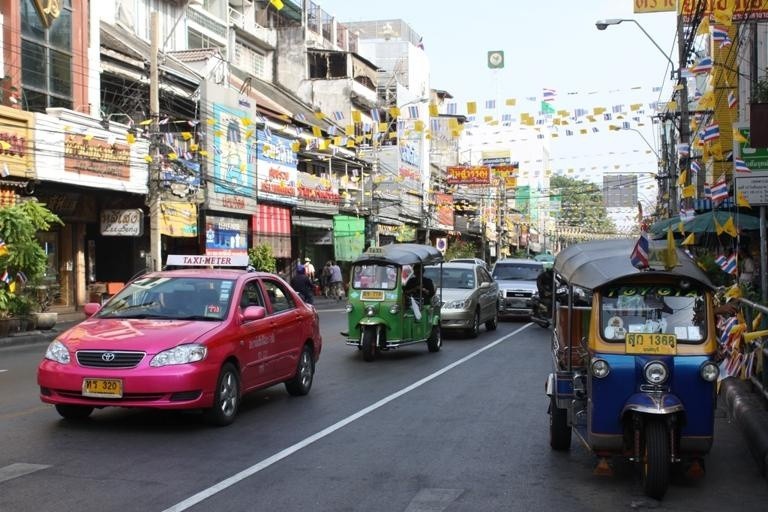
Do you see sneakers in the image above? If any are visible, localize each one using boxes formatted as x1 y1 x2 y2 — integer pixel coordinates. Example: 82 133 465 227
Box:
323 292 342 300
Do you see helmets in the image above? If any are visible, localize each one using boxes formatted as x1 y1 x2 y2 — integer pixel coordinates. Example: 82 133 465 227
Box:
297 266 306 275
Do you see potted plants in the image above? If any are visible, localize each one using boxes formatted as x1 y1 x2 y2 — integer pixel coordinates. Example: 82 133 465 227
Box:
27 270 63 330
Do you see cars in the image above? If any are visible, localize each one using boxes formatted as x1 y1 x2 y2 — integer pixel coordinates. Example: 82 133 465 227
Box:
446 257 488 274
420 262 499 343
35 253 322 431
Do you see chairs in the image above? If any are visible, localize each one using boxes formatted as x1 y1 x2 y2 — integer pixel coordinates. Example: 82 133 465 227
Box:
257 288 277 312
221 286 251 322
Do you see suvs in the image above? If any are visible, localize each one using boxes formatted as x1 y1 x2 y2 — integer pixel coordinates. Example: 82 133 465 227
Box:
490 257 548 324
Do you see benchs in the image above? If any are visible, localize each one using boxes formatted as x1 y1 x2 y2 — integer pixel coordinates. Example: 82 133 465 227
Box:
552 303 660 377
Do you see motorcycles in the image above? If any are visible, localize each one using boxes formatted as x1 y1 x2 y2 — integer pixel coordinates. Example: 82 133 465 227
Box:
340 239 451 365
522 297 552 333
542 236 723 501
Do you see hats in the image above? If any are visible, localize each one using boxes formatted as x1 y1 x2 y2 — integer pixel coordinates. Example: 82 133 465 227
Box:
304 257 313 263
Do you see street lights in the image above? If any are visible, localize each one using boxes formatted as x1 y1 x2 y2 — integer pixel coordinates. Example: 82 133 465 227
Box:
595 18 694 216
370 94 433 246
480 172 519 258
612 124 669 215
